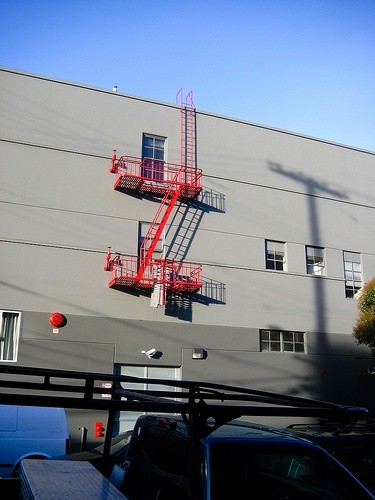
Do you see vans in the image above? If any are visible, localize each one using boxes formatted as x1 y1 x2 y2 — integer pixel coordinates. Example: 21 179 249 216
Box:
0 404 69 478
118 415 375 500
